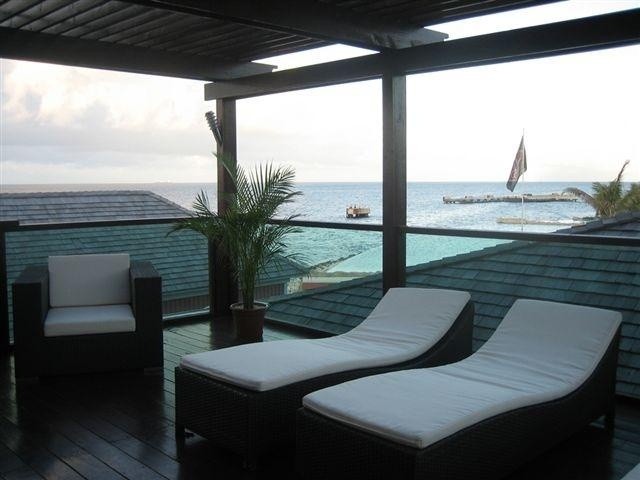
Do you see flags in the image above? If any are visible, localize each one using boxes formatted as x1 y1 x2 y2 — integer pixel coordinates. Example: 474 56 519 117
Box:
504 137 528 193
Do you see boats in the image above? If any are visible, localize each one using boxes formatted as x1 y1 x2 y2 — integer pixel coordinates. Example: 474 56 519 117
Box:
346 204 370 218
442 191 578 205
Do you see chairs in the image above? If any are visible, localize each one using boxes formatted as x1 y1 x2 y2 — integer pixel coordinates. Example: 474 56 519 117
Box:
174 288 472 480
11 253 163 416
298 299 623 480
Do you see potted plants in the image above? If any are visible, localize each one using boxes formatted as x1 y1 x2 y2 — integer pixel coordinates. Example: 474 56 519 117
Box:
164 155 312 344
